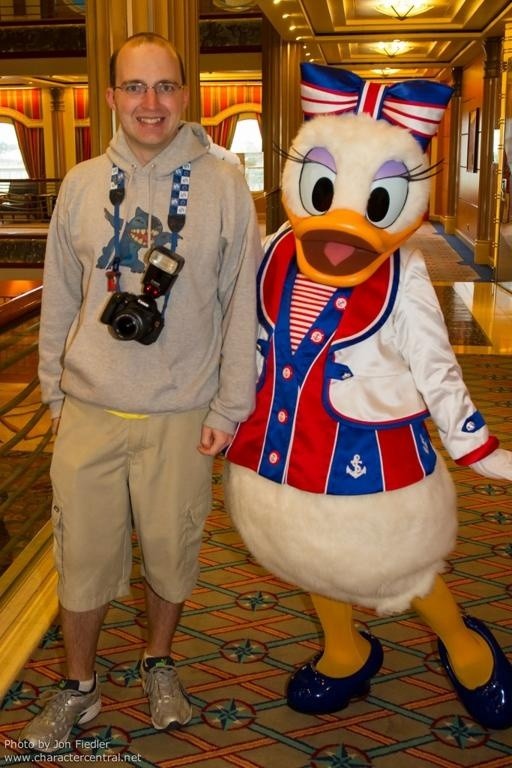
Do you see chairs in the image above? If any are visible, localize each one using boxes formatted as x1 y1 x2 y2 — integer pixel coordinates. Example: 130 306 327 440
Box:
0 178 41 224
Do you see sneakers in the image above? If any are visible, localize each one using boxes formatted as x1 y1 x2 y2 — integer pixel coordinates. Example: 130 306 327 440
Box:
139 659 192 731
19 672 101 753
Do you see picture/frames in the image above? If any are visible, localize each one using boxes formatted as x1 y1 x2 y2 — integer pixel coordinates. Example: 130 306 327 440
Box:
466 107 479 173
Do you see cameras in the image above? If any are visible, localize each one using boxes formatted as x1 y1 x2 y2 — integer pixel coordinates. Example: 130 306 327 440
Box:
98 247 185 345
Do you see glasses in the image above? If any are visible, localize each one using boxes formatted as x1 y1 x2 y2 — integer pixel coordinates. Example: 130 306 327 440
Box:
113 83 184 97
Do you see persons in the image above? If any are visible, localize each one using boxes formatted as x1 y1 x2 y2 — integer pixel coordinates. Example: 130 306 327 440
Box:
16 32 259 759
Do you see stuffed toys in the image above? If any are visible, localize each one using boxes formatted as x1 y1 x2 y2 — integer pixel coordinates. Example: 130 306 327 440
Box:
224 63 512 732
